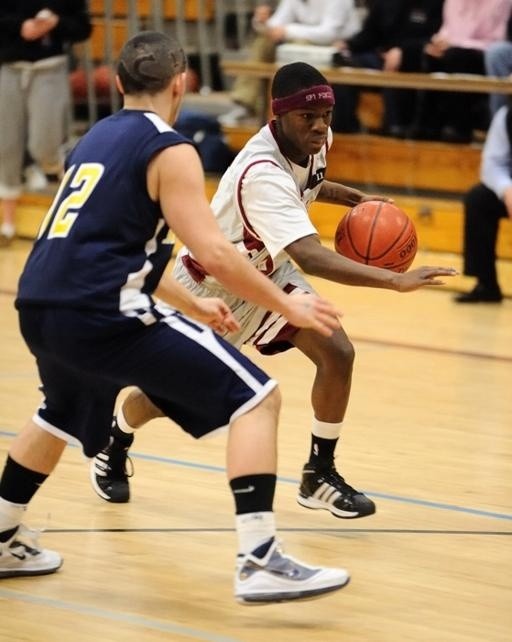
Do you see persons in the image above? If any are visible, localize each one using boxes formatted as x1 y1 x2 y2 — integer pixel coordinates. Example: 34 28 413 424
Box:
332 1 444 139
217 1 288 127
90 60 458 520
251 1 363 63
0 29 349 604
391 1 510 142
452 107 512 305
0 0 93 249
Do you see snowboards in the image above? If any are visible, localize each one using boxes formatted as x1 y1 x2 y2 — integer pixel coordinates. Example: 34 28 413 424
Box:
335 200 418 272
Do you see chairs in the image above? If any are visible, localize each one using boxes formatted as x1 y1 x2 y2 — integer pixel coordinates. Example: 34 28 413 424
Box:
456 284 502 302
297 464 375 518
233 536 349 599
0 526 62 578
91 415 133 502
